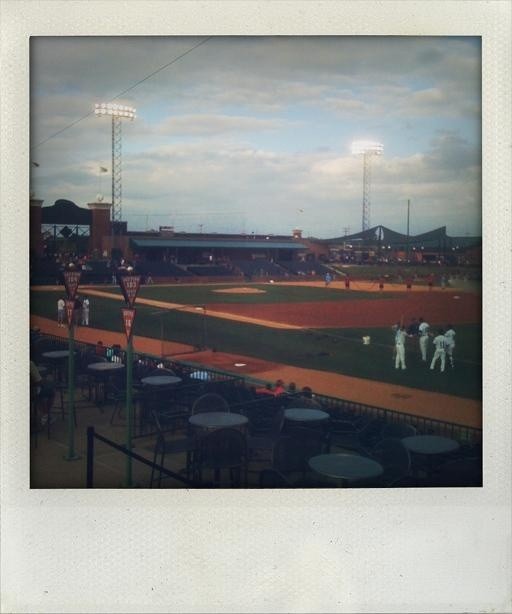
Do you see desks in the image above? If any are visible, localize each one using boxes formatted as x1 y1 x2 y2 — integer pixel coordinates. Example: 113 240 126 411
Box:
274 408 463 488
31 336 78 449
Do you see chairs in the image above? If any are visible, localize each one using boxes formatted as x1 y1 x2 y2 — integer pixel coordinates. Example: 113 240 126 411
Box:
136 368 249 489
82 354 127 424
